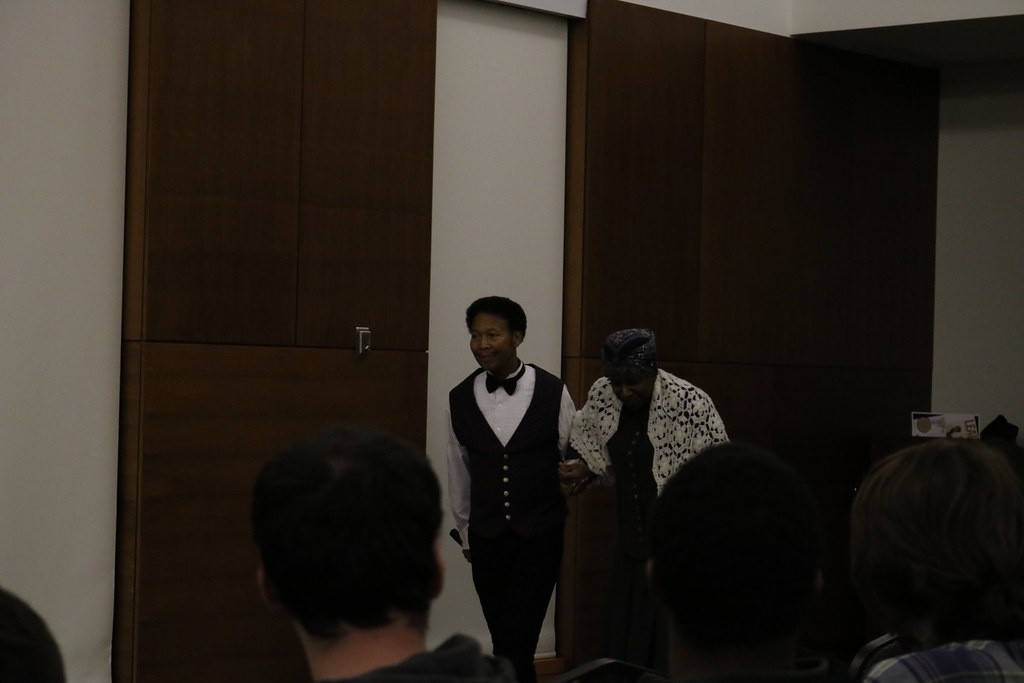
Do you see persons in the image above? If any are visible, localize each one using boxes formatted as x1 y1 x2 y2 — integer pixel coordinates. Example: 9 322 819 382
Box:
557 327 732 668
640 442 850 683
247 427 517 683
445 295 590 683
848 438 1023 683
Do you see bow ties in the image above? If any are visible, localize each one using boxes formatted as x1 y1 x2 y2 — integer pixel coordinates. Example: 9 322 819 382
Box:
485 361 526 396
